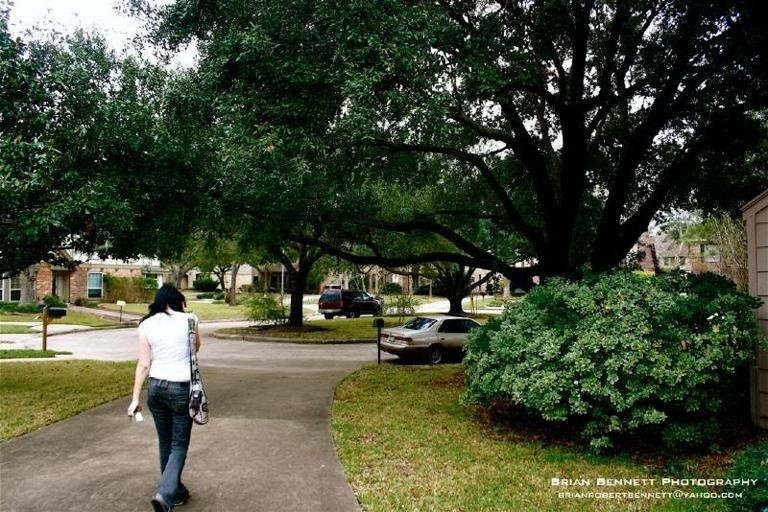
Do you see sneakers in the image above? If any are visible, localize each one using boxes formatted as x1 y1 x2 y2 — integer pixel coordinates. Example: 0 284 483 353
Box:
150 492 174 512
172 489 189 506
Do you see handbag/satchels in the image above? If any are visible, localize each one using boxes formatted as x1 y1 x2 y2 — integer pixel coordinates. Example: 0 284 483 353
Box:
186 312 208 425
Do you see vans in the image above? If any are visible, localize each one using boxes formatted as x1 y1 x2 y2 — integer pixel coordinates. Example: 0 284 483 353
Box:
318 288 383 317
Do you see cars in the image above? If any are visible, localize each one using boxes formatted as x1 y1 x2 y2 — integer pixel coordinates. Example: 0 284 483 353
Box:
379 316 481 365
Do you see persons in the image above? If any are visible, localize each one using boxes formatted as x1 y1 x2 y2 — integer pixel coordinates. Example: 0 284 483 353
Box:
127 282 201 512
